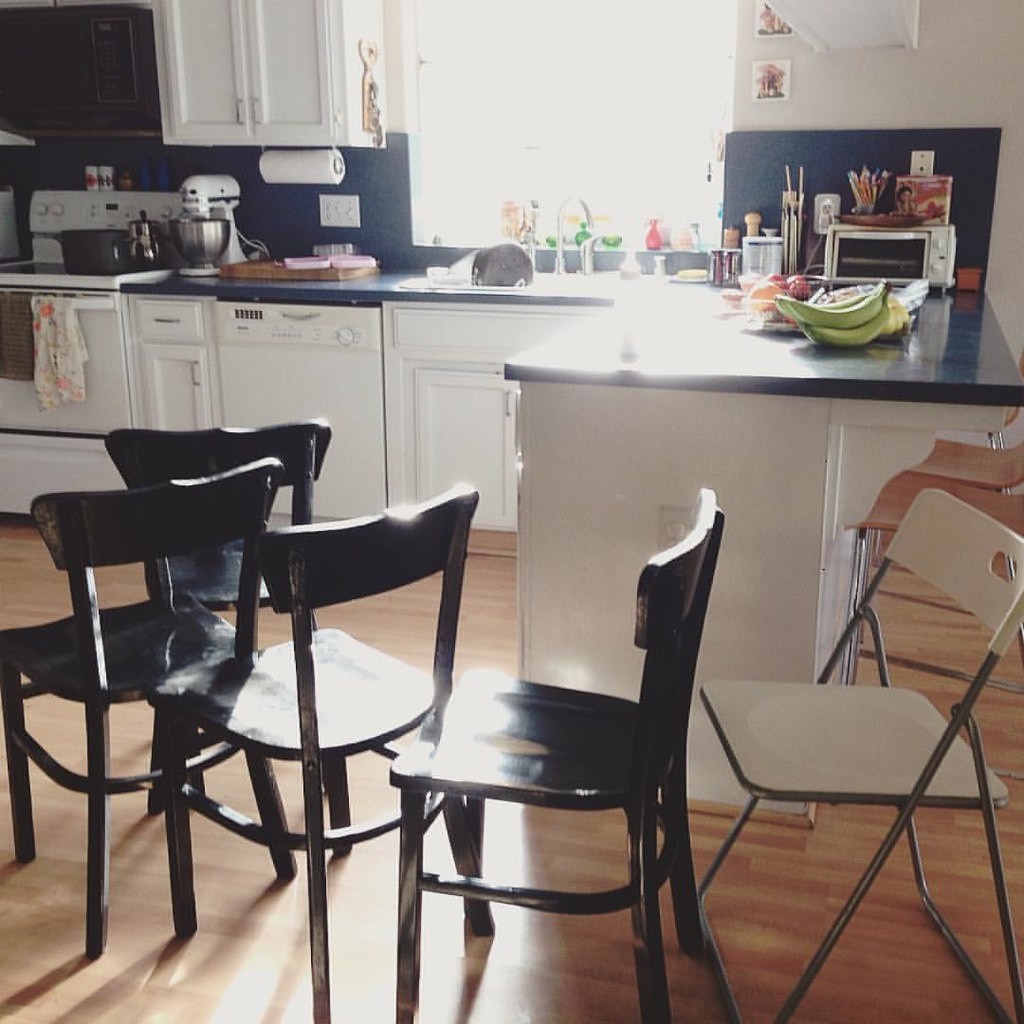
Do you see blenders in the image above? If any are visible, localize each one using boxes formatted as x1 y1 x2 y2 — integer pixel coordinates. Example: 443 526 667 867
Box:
179 173 250 275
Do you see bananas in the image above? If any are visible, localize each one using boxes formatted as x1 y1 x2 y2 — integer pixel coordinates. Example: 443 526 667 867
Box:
773 278 892 351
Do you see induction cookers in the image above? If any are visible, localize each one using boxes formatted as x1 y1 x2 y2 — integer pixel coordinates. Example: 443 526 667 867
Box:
0 190 181 291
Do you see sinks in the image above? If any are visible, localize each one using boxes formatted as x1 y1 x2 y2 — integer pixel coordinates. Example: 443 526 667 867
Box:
399 260 622 309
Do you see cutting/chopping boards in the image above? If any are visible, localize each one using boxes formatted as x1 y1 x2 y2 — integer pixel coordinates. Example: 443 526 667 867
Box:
220 260 376 281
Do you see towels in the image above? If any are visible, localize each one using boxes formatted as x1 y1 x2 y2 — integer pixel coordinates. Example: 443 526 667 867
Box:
0 292 36 384
30 290 90 413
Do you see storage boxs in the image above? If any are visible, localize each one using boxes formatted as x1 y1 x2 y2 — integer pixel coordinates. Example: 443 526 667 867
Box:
894 175 952 224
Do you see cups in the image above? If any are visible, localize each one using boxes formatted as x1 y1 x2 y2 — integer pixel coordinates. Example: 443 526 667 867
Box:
709 248 741 284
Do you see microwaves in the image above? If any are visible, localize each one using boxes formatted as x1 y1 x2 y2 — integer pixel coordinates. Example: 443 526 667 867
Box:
0 5 161 133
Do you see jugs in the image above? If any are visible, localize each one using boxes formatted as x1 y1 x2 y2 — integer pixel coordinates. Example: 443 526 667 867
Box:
122 210 169 269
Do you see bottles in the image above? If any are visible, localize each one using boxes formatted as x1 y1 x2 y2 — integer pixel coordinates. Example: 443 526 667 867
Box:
576 222 592 246
646 219 661 250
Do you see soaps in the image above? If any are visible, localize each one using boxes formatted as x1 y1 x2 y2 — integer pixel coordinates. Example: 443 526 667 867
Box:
677 267 710 282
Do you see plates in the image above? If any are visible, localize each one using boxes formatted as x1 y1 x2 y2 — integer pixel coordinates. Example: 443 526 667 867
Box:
666 275 707 283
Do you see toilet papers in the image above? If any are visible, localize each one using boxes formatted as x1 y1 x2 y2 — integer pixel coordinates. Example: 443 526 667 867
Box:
258 146 347 186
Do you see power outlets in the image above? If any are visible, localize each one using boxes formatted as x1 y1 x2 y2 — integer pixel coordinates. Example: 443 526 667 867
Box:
319 194 359 228
814 193 841 235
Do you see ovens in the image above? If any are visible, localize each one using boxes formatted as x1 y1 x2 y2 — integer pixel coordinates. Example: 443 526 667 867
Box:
0 283 131 434
824 223 956 292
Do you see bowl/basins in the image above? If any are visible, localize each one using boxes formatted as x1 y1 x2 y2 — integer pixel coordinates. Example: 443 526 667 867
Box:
61 230 130 274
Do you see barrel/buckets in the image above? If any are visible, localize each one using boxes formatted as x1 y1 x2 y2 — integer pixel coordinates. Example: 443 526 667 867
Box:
168 219 231 268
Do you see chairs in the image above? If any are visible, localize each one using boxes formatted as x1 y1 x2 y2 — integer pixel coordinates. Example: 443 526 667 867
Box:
0 456 297 960
106 419 333 794
698 488 1023 1024
906 438 1024 489
390 490 725 1023
844 473 1024 697
148 480 483 1024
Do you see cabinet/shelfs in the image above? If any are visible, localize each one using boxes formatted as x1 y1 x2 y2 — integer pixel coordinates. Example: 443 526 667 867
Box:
150 0 385 148
380 303 610 532
126 295 212 433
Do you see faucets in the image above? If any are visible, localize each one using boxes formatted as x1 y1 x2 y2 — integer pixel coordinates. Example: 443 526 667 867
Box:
549 195 595 278
